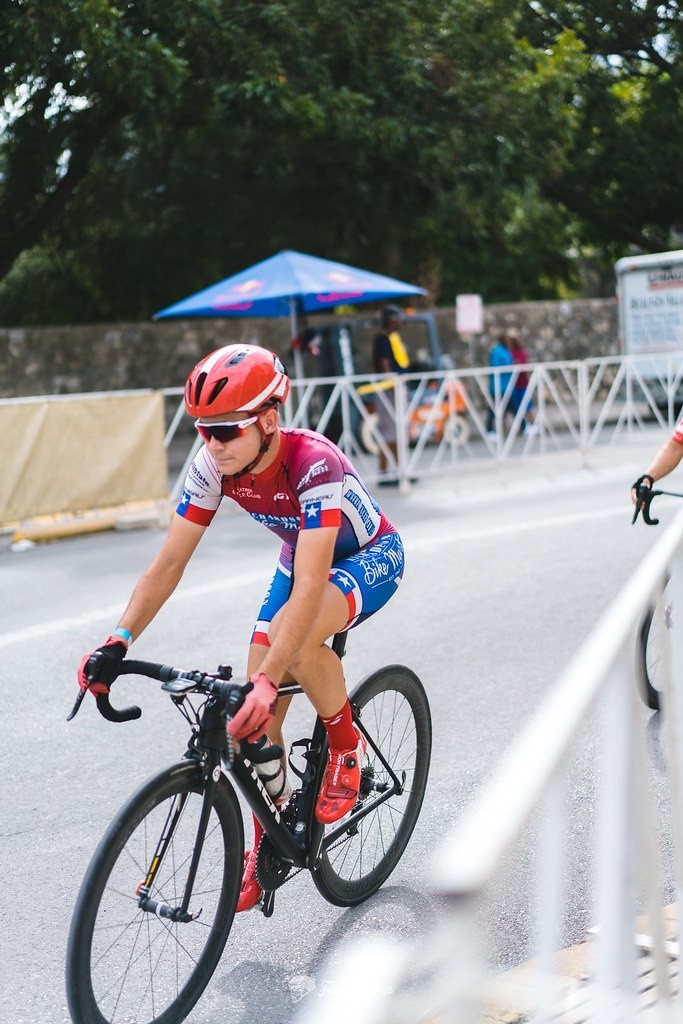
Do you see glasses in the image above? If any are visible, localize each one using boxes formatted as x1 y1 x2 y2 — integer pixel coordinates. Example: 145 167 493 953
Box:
194 402 279 443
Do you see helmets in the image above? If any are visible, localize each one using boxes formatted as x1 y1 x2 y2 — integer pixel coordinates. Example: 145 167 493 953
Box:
184 343 290 417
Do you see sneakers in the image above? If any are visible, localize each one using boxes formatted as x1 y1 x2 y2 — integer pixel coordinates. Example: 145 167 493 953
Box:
235 850 260 913
315 726 368 823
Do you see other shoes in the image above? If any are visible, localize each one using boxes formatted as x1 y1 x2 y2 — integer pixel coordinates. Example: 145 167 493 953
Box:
487 431 498 441
378 477 419 488
523 423 540 437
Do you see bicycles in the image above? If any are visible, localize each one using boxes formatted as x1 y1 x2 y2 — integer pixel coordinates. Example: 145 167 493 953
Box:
64 631 434 1024
631 474 683 711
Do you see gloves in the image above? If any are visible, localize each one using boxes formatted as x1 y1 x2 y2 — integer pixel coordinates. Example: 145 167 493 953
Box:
226 671 279 749
77 636 128 698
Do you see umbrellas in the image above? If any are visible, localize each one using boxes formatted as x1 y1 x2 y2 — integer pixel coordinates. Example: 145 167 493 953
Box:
152 250 430 429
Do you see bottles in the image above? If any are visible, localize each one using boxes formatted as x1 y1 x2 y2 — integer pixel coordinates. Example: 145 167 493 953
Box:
249 733 293 806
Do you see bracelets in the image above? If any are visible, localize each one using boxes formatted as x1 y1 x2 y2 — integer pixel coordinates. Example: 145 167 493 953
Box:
112 627 132 647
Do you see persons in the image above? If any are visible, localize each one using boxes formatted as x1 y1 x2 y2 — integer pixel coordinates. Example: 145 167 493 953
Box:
77 343 404 913
631 415 683 510
371 306 419 486
485 333 537 443
